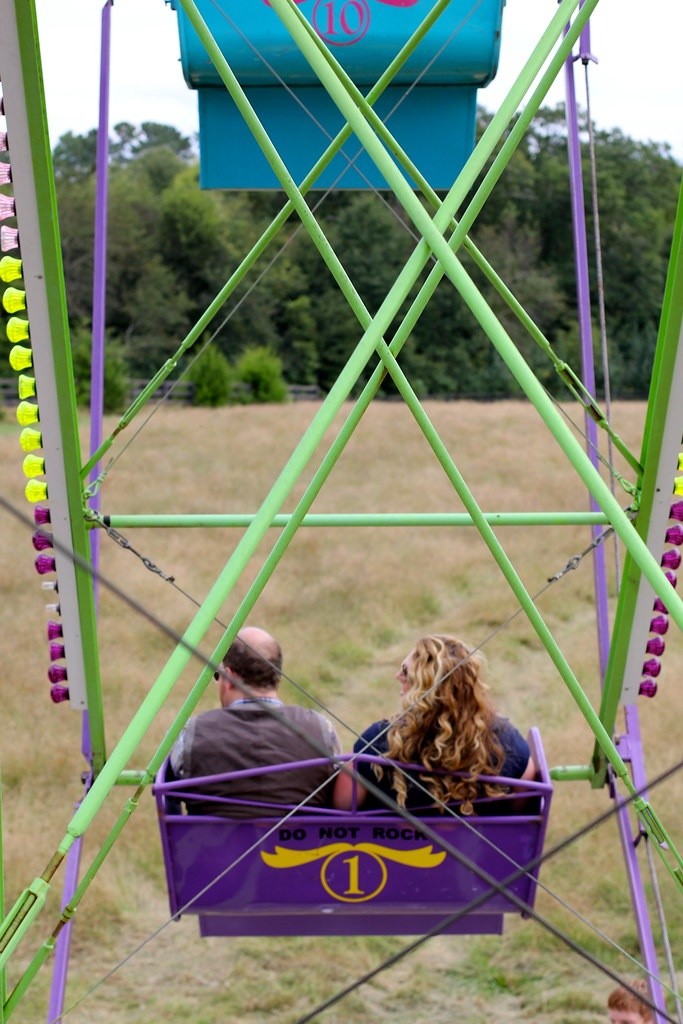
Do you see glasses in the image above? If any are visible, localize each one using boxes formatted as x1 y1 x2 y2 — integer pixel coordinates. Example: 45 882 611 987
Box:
400 662 408 677
214 672 220 681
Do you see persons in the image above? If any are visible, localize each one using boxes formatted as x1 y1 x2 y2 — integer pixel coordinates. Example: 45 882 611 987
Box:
333 635 535 816
170 626 342 819
